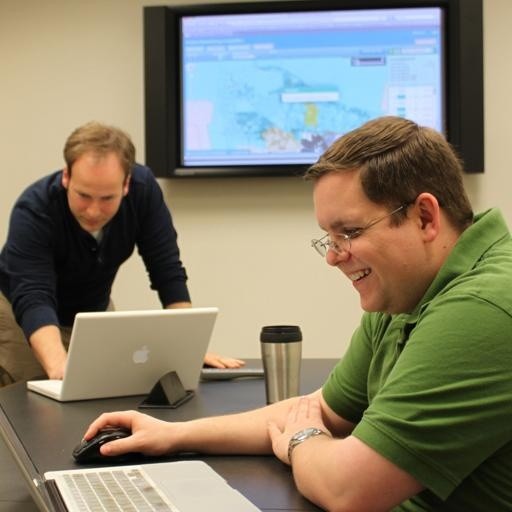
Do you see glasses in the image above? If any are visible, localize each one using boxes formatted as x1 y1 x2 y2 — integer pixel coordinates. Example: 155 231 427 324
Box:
311 203 406 259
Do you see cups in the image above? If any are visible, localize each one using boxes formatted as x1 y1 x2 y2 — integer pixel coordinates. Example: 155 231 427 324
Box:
259 324 302 405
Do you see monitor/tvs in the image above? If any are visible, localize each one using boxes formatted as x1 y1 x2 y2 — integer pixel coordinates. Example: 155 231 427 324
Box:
143 0 485 179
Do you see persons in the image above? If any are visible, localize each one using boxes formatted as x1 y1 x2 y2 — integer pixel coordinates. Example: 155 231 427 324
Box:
80 116 510 511
0 120 247 389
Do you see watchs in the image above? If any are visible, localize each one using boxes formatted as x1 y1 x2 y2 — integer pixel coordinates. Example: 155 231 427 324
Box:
287 428 331 463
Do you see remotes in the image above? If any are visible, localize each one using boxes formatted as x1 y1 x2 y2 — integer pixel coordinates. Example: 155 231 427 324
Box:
200 368 264 382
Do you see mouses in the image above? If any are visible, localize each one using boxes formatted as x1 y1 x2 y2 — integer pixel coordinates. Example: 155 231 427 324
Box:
71 429 142 463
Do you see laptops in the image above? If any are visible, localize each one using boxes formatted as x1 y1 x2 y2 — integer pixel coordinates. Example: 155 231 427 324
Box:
26 305 218 402
0 405 260 512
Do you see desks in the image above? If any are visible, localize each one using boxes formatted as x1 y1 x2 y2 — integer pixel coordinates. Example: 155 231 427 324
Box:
0 358 343 512
